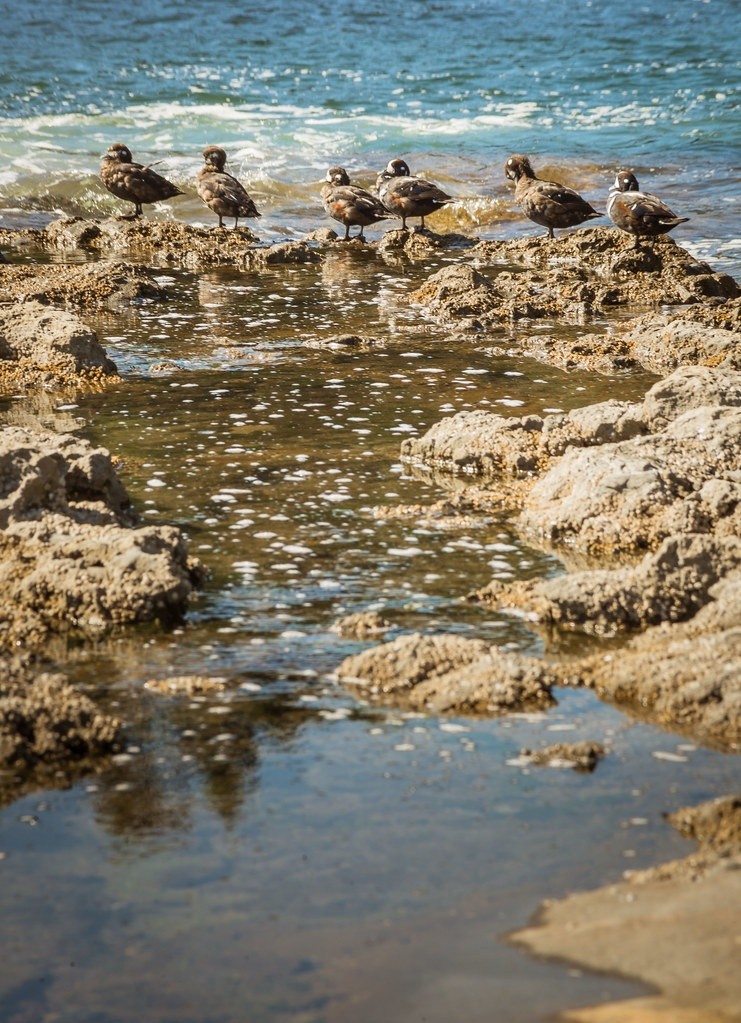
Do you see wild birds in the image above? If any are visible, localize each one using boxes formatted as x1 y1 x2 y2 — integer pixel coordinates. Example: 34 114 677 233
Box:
373 158 456 234
607 170 690 251
320 167 395 243
196 146 262 231
504 153 606 241
100 143 186 220
